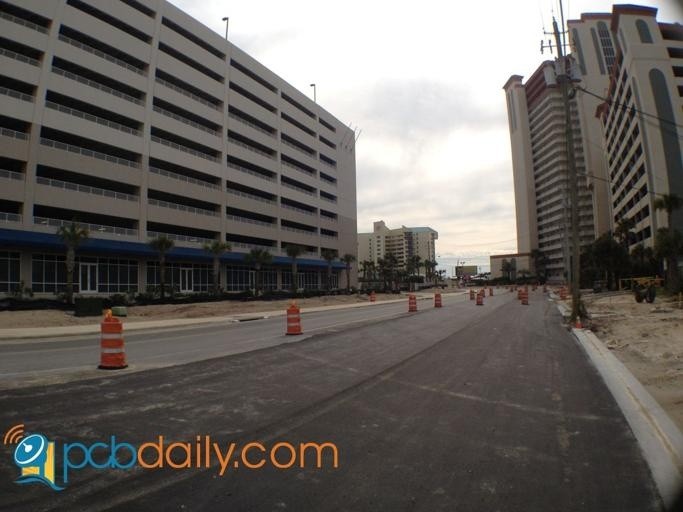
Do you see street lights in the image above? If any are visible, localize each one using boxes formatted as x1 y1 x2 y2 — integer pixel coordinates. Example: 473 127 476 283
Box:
220 15 233 41
308 81 319 103
